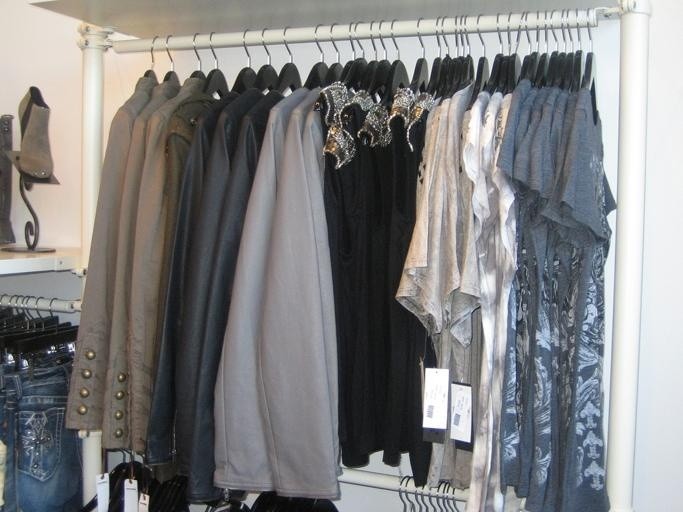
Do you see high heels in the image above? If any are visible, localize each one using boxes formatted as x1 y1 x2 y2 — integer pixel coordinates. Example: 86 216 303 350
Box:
18 86 54 179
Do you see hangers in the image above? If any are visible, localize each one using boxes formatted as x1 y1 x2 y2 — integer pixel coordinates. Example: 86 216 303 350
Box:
76 450 188 512
150 6 605 148
0 294 78 358
